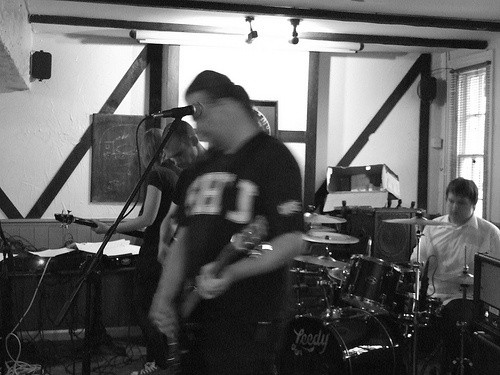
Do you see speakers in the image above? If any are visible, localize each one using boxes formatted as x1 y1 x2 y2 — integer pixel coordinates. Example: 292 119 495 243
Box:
31 50 51 81
474 250 500 339
372 208 426 265
30 52 51 79
421 76 437 98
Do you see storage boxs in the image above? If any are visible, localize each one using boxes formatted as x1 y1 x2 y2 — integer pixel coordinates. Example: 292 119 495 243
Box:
323 164 402 212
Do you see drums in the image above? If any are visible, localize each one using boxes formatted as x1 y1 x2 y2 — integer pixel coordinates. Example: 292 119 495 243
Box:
391 260 422 319
327 267 350 308
275 306 397 375
340 254 404 316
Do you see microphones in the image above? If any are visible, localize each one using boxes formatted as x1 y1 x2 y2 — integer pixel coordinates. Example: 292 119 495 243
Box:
150 103 203 119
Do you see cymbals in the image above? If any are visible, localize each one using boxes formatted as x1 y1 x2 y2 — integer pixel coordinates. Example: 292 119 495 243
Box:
433 271 474 286
302 231 360 244
303 212 347 224
292 255 347 268
382 216 444 226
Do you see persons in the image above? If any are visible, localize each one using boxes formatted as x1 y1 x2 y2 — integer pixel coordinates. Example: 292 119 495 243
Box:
410 176 500 302
92 71 307 375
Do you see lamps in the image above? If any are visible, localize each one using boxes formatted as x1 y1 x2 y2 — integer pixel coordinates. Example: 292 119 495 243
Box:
289 17 300 45
244 16 258 44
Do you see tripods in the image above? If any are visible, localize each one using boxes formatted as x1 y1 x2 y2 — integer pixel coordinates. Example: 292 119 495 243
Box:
447 284 476 375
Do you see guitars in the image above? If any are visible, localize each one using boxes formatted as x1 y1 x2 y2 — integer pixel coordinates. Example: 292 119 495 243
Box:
120 215 270 375
55 210 145 239
